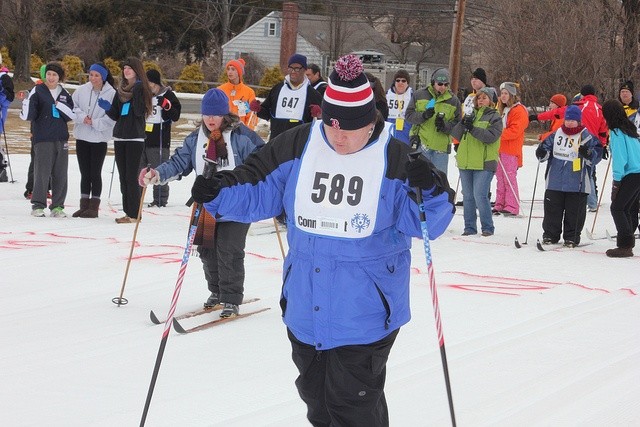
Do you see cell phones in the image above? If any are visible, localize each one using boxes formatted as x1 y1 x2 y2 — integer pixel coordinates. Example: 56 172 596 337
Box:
436 111 445 119
465 112 472 120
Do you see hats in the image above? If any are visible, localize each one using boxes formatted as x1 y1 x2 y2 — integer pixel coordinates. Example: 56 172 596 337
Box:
470 67 486 84
89 62 108 82
322 54 377 130
480 87 499 103
550 94 567 107
288 54 307 70
146 70 160 86
394 70 410 84
500 81 520 95
581 85 595 95
564 105 582 124
226 58 245 80
620 81 634 93
202 89 229 115
432 68 450 82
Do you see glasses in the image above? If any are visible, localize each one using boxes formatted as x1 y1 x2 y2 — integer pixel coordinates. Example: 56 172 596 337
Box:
395 80 409 82
438 82 450 86
289 66 303 72
204 114 225 119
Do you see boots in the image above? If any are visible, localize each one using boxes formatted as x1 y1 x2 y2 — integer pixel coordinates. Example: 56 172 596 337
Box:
80 198 101 217
72 198 90 217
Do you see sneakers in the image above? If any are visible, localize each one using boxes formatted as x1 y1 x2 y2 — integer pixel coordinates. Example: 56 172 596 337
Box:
563 240 577 248
492 208 498 213
542 238 558 244
587 207 597 212
207 292 220 306
26 192 33 199
462 232 477 236
0 169 8 182
50 206 67 217
31 209 45 216
494 210 516 217
481 232 493 236
116 215 141 223
220 302 239 318
47 192 52 198
148 201 165 207
606 246 634 257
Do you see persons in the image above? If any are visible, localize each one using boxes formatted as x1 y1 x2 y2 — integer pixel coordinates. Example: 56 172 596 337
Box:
0 52 15 182
456 67 499 206
455 87 504 237
602 99 640 258
138 88 267 319
536 94 568 142
529 85 609 213
495 81 529 217
618 80 640 137
404 67 464 176
305 63 328 96
71 62 117 219
248 53 323 227
19 61 74 217
97 56 153 223
216 58 259 131
145 69 182 209
535 104 603 248
17 64 53 200
384 70 415 145
191 52 457 427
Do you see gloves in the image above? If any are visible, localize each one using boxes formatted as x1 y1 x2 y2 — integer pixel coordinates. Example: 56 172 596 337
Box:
156 96 169 108
529 115 537 121
435 112 445 126
462 115 472 125
136 79 143 84
98 98 112 111
579 143 593 159
423 107 435 120
602 146 609 159
250 99 261 112
192 175 219 204
139 167 158 187
309 105 322 117
536 146 547 157
612 181 621 201
464 117 473 131
405 159 434 190
